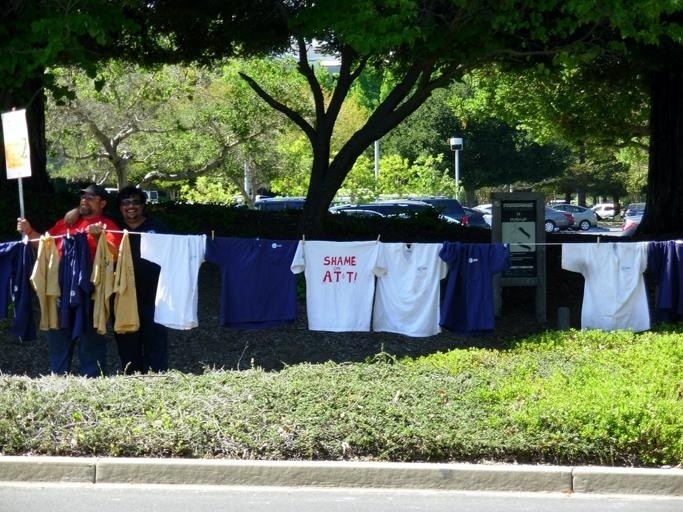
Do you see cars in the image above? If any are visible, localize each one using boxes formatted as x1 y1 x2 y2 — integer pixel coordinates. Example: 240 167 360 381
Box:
545 204 574 233
464 204 492 228
591 204 624 219
624 202 647 224
552 204 596 230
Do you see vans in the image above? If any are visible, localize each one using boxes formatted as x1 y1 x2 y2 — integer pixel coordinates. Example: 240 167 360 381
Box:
236 200 305 210
331 199 468 226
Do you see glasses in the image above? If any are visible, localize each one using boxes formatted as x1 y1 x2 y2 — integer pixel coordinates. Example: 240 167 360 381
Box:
81 195 103 200
120 199 143 205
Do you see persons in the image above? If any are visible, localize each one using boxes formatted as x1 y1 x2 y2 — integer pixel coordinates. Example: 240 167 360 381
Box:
63 185 170 374
16 184 122 378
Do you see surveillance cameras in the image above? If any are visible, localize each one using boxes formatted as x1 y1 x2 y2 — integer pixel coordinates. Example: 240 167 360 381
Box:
450 138 463 151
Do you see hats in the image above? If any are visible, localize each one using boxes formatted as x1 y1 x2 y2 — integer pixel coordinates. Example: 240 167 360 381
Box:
80 184 108 200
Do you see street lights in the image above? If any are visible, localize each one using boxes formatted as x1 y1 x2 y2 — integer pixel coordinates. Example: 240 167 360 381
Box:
450 138 463 186
